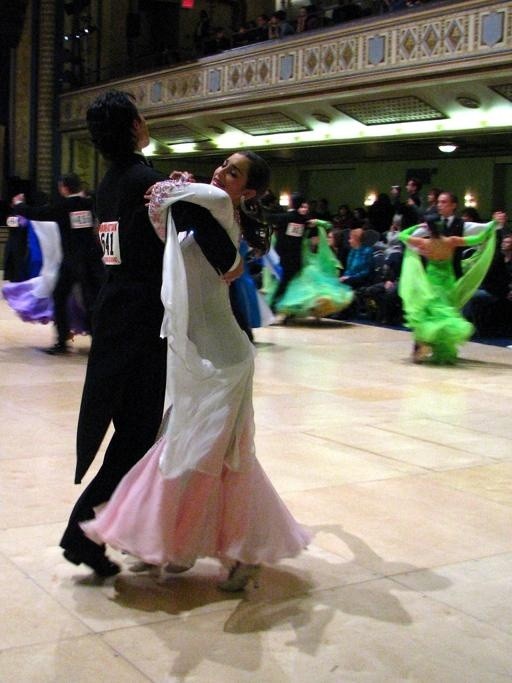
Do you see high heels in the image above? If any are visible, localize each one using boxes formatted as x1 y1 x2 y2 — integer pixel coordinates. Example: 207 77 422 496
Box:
219 562 262 595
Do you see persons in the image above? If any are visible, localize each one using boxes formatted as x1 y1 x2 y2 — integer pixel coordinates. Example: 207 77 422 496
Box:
58 91 243 577
78 150 316 591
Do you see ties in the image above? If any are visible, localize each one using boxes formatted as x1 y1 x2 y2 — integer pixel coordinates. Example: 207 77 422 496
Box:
441 217 449 230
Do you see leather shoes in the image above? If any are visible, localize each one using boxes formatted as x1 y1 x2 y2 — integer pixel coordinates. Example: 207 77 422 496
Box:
63 547 122 577
